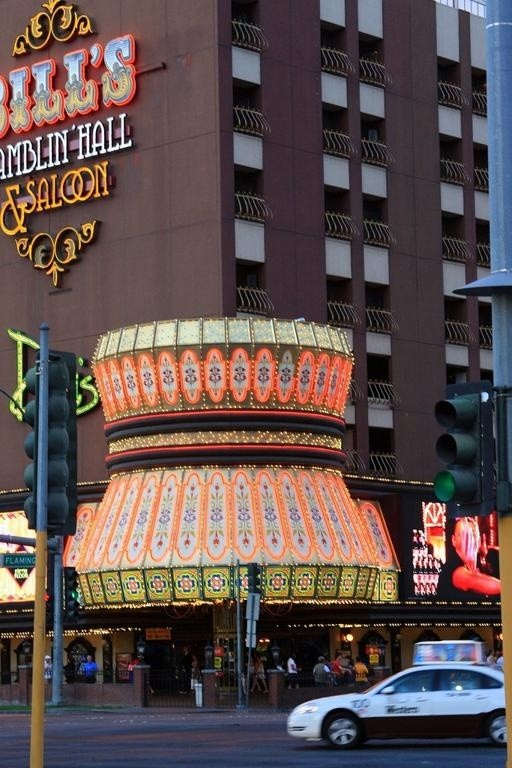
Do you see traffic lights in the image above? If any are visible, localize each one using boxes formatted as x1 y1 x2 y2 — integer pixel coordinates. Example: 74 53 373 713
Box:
64 565 80 624
247 563 262 594
45 592 54 631
432 379 500 519
21 358 71 531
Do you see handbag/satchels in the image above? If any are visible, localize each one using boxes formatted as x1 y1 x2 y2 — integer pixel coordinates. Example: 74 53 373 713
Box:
191 679 198 689
256 672 266 680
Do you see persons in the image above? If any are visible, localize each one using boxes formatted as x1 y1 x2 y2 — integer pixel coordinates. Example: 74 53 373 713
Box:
176 645 302 695
43 651 154 696
484 645 504 674
413 514 500 597
312 651 369 688
367 648 384 664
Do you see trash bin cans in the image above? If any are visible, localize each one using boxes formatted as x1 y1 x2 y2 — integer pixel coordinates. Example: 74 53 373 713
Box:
195 684 202 707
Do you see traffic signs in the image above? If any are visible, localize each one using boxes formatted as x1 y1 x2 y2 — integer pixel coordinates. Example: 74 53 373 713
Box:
0 553 38 568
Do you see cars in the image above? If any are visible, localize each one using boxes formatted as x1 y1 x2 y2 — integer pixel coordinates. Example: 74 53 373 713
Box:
283 661 508 750
